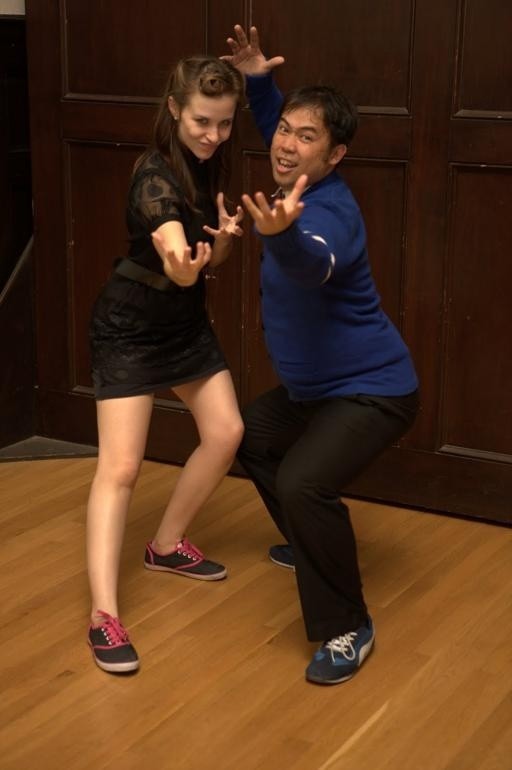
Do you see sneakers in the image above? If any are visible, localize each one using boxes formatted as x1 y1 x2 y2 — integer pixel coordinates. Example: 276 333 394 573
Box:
269 543 296 570
143 534 228 582
86 617 140 673
305 613 375 685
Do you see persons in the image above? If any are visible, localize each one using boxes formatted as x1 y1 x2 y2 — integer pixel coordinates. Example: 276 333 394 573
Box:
86 55 250 674
220 23 421 685
